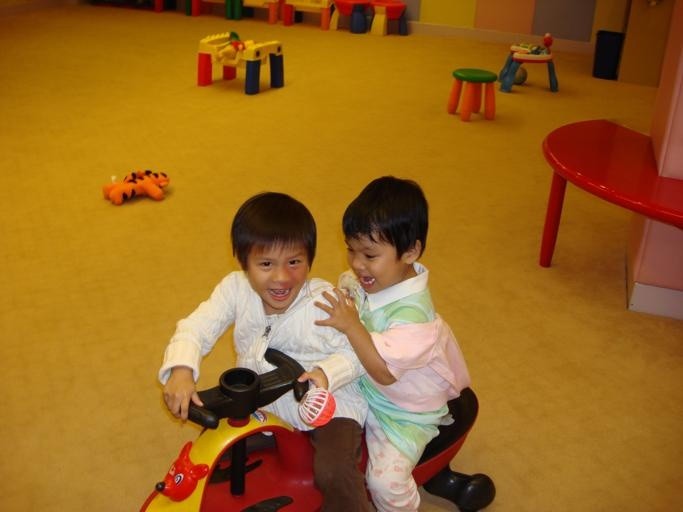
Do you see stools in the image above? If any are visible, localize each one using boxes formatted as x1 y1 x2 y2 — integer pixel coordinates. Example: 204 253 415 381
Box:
445 68 499 125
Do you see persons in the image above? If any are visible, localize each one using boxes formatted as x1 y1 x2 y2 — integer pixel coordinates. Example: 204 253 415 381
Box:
313 174 470 510
157 191 373 510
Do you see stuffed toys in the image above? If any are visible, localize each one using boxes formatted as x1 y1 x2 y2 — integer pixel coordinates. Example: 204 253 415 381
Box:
103 169 170 205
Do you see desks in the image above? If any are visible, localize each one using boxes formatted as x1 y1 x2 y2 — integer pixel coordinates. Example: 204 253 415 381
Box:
539 117 682 281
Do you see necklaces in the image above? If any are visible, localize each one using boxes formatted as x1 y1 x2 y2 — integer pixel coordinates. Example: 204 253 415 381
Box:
260 307 289 339
